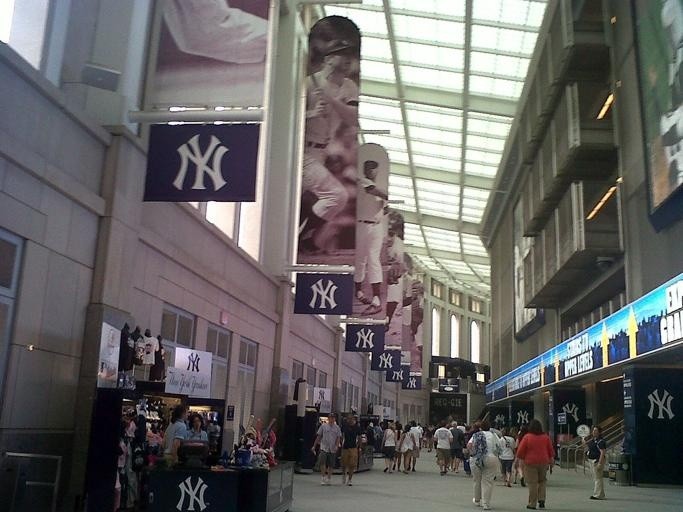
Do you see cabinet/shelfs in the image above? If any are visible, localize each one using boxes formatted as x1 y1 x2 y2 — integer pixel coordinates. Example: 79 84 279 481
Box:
80 388 227 512
151 457 296 512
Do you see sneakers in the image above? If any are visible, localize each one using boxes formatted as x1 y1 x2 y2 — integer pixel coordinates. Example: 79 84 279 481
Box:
473 497 481 505
527 505 537 509
361 304 382 315
384 463 415 474
356 291 372 304
483 503 492 509
540 501 544 507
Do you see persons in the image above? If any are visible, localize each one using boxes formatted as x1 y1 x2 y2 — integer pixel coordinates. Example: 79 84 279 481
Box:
119 322 135 372
185 413 209 450
516 418 556 510
162 0 268 64
466 420 502 511
387 220 403 330
142 329 159 365
402 255 411 355
149 334 166 381
582 425 608 502
131 325 145 366
358 159 387 314
298 39 357 255
145 344 151 354
312 407 526 489
412 279 423 373
162 404 188 467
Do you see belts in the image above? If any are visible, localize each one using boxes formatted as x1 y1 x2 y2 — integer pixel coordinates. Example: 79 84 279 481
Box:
307 140 329 149
358 219 375 224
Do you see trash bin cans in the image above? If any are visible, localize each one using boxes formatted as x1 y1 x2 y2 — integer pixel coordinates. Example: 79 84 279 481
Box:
608 453 631 486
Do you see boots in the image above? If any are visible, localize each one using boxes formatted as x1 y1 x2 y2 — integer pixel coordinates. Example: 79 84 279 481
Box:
301 210 326 254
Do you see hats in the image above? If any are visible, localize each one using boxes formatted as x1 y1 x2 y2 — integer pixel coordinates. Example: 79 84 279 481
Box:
364 159 377 167
325 38 353 55
